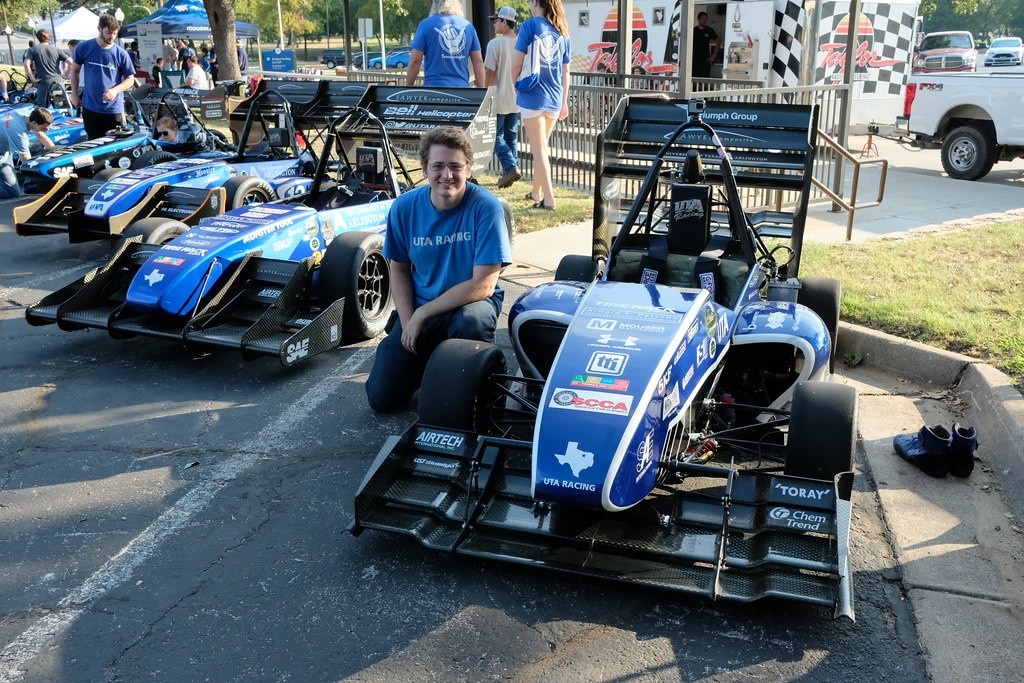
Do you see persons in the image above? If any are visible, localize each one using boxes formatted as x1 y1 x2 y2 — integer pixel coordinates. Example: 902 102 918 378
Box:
23 29 249 109
692 11 720 92
483 6 521 189
156 117 195 143
364 128 513 413
0 76 11 101
0 108 56 200
405 0 486 89
511 0 572 208
71 15 136 175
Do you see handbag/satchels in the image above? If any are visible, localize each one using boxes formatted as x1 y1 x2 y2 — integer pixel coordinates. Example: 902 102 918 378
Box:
514 18 540 94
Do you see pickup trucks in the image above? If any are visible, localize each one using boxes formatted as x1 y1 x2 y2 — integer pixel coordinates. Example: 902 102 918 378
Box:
896 72 1023 182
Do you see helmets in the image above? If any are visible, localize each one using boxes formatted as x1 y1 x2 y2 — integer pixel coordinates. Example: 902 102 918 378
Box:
26 87 38 97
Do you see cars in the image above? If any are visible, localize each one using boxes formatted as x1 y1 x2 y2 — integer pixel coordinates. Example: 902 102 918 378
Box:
354 52 386 70
984 37 1024 66
388 47 412 55
368 53 399 70
389 52 412 69
911 31 986 74
320 50 355 69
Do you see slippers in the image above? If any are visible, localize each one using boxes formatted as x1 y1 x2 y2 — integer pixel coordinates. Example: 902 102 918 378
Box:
524 192 535 201
531 199 556 211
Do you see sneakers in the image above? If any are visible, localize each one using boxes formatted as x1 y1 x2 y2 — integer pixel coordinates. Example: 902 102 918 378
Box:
496 168 521 188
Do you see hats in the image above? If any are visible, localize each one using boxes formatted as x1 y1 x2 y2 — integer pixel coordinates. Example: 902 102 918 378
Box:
488 6 516 23
236 40 244 47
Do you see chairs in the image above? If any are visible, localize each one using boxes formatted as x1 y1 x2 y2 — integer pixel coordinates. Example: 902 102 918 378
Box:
309 187 389 211
612 250 750 311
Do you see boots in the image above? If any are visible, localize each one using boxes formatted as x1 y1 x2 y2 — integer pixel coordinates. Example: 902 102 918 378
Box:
893 422 980 479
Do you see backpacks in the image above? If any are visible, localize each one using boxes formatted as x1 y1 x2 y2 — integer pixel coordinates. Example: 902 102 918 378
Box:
188 47 196 57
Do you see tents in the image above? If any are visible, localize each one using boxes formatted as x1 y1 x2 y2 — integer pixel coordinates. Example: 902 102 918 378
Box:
117 0 263 75
34 6 101 40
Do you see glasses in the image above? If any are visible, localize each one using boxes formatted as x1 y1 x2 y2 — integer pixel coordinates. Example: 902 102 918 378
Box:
425 161 467 172
176 44 180 48
156 131 169 137
